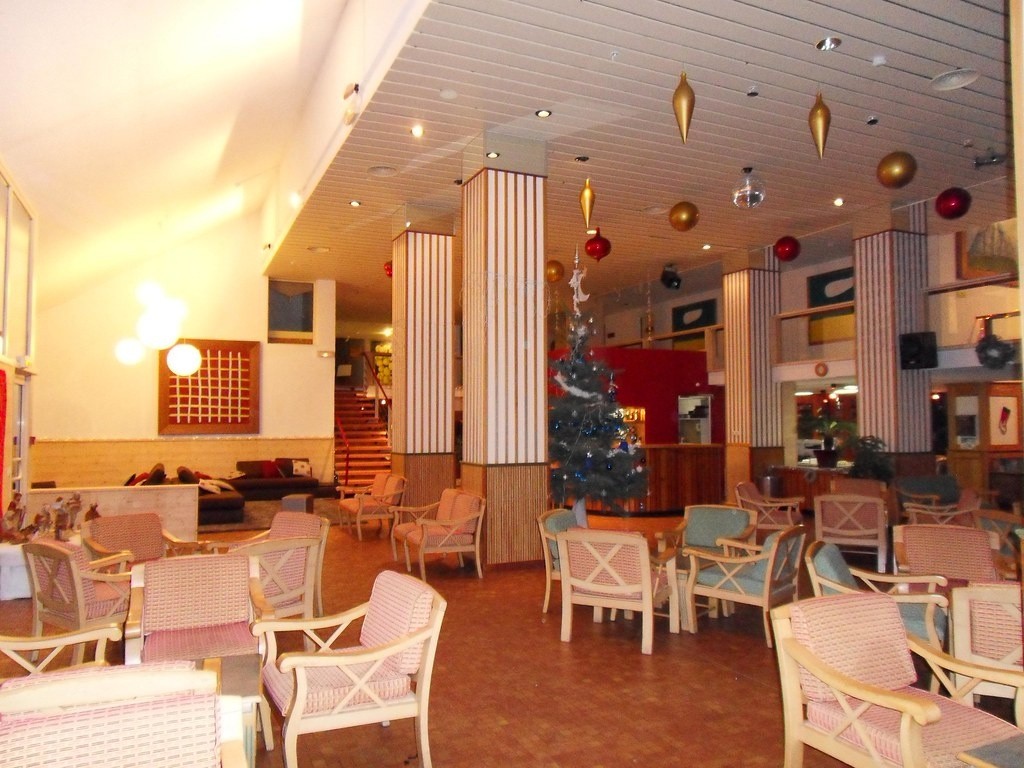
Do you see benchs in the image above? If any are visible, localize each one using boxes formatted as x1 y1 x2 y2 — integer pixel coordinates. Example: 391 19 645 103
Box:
141 463 165 486
388 489 487 583
336 473 407 541
171 466 245 524
230 458 319 500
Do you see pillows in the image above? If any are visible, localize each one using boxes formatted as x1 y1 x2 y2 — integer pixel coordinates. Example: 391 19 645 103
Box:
195 471 212 480
129 473 149 486
293 460 310 476
124 473 136 486
199 478 234 494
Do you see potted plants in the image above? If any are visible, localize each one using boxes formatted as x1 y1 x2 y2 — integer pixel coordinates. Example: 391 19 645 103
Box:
797 405 857 468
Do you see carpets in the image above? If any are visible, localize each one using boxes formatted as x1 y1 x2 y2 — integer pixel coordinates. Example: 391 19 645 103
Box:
198 498 368 534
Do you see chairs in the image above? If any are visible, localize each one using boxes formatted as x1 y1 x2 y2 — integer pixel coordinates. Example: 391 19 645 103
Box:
0 512 447 767
537 478 1024 767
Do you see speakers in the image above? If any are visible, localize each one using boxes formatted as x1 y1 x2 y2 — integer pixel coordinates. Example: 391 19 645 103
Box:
899 332 937 370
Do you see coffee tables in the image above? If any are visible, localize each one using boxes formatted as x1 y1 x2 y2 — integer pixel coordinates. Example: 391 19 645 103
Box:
282 492 314 514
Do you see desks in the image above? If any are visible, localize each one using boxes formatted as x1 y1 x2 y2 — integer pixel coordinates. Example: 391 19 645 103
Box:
190 654 263 768
957 735 1023 768
775 466 847 510
650 549 717 631
0 535 81 601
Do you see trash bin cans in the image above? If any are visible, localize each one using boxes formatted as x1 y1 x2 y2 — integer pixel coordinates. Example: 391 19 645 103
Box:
758 475 783 499
